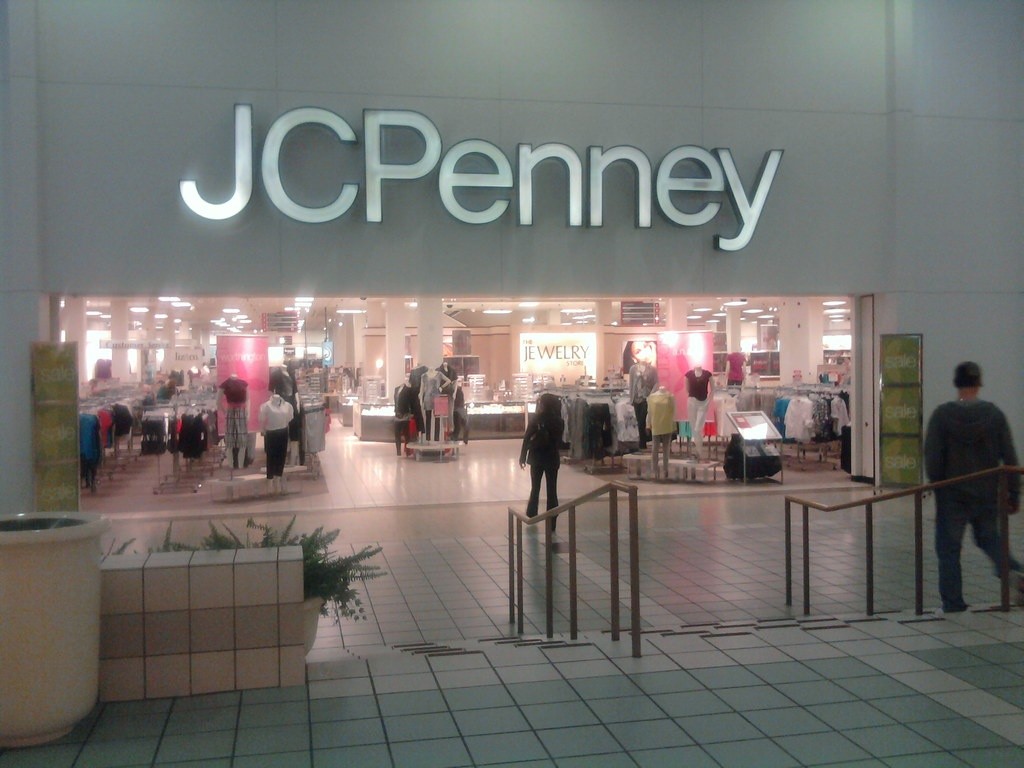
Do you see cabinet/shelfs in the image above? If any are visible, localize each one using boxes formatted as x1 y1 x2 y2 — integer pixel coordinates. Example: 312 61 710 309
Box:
79 351 852 494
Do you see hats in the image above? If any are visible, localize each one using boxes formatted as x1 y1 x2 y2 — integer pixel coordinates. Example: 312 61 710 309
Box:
953 362 987 388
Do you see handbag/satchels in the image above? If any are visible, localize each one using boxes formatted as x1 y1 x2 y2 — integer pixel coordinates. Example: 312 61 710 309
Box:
528 429 555 457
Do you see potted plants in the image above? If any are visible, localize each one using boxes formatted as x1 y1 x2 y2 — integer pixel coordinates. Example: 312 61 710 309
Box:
144 519 388 660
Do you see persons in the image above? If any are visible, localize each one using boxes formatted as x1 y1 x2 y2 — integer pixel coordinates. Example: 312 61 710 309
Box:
259 395 294 496
393 359 469 456
268 364 300 469
925 361 1023 612
646 386 677 480
141 376 176 408
188 366 200 385
171 367 185 386
626 357 660 452
216 374 250 469
519 393 565 530
684 363 717 464
723 347 747 391
623 341 657 374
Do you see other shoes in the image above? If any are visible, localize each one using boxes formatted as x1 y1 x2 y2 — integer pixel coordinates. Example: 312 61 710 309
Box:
1006 570 1024 607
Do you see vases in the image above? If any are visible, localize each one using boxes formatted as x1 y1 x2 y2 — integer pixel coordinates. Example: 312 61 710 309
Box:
0 511 105 749
